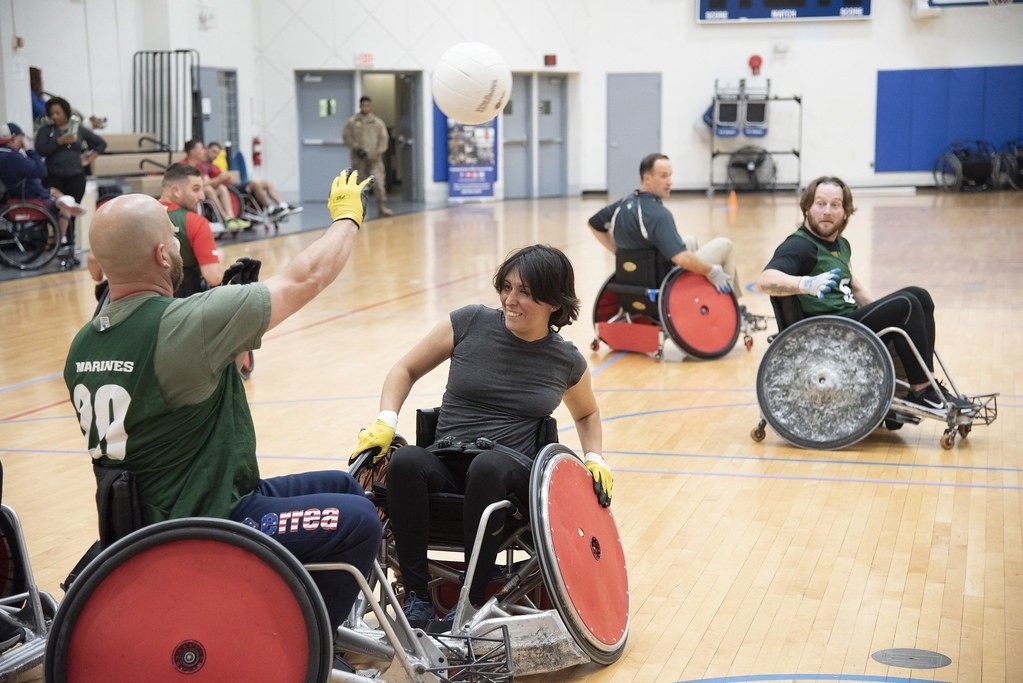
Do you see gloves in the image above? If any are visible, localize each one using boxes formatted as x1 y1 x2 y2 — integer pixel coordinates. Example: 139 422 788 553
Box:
583 452 615 507
347 418 395 465
327 167 375 230
219 258 262 288
799 267 842 300
705 264 731 294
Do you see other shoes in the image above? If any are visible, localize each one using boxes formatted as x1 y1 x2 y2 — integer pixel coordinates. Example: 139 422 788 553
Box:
58 243 81 255
379 208 392 217
280 202 303 213
55 195 86 216
739 309 766 331
226 217 252 230
268 207 288 220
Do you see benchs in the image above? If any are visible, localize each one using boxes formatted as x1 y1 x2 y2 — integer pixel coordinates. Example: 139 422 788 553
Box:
82 131 188 199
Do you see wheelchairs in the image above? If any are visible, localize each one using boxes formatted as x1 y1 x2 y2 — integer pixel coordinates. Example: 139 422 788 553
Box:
0 176 83 273
138 136 246 241
341 409 628 679
37 458 519 683
934 138 1023 193
0 503 62 682
232 186 292 238
592 262 769 362
746 283 1000 451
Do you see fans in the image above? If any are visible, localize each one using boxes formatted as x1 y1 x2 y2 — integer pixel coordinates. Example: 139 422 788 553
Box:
725 145 777 192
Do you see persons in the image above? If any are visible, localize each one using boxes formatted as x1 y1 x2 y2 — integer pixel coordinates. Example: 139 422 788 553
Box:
587 153 743 300
64 166 383 672
235 180 303 217
758 177 979 413
35 97 107 268
342 95 393 215
0 122 86 247
159 162 224 292
349 243 614 631
179 140 251 230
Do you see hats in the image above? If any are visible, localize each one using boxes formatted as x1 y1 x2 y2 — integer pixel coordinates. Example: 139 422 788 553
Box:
0 122 23 144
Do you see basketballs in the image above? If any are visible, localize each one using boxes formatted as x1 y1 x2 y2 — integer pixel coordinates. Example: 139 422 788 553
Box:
428 41 513 125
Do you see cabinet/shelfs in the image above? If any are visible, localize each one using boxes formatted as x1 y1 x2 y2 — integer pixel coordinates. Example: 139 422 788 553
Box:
707 96 802 197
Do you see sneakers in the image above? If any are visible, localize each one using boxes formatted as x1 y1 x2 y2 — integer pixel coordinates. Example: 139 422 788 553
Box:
400 590 435 631
906 378 978 415
445 601 457 620
